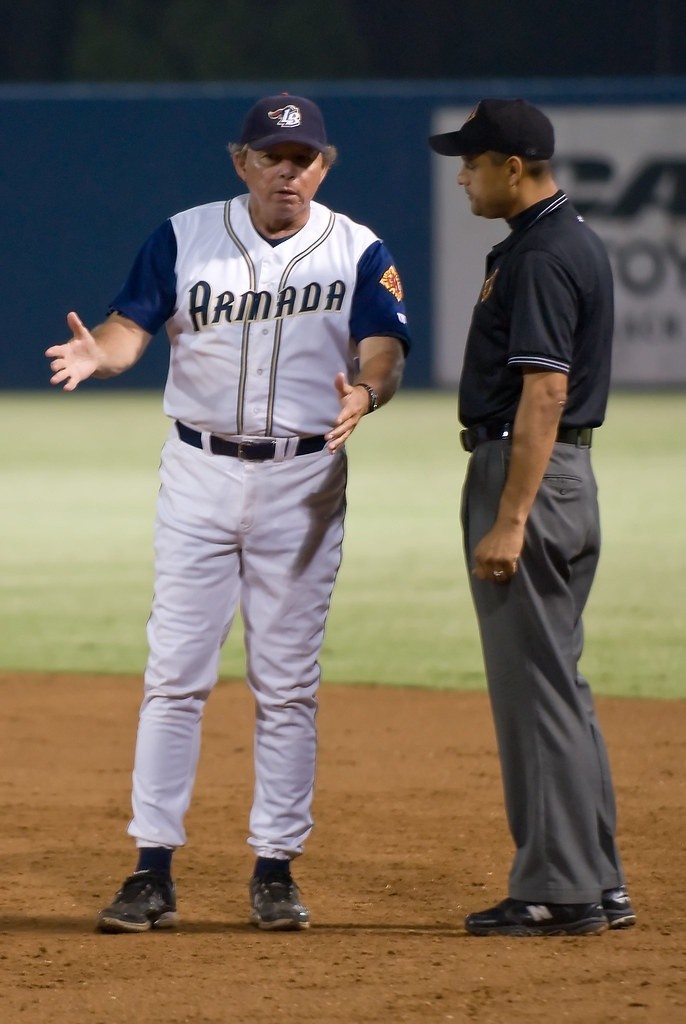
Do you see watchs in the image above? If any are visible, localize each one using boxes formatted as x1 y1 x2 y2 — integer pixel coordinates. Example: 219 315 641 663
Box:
353 383 377 415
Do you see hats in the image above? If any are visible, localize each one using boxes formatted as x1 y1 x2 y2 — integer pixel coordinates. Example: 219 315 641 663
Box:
428 99 556 160
240 93 327 154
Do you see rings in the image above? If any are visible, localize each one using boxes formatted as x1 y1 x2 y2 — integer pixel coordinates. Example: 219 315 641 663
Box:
492 569 504 579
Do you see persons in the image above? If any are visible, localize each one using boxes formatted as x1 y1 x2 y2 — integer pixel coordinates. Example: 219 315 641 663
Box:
427 95 637 940
42 93 412 936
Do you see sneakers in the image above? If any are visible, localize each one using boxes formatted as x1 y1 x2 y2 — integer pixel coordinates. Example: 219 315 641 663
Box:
601 885 637 929
465 897 609 936
249 872 309 930
99 870 177 931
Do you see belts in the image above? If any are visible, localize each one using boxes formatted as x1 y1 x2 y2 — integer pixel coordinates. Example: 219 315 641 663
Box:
176 420 328 462
460 420 592 452
